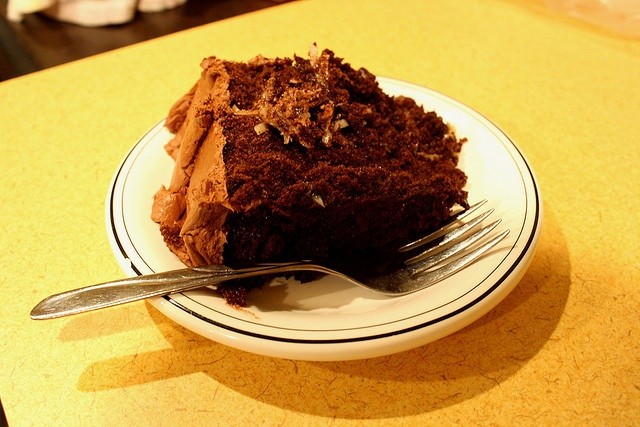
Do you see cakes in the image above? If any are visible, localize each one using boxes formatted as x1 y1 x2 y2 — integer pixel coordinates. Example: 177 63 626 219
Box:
152 41 469 303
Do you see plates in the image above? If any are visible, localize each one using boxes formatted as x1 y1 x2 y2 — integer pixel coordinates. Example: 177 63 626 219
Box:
105 77 543 361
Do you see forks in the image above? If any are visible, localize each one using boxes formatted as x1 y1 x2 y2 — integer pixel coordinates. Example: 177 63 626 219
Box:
29 198 511 321
